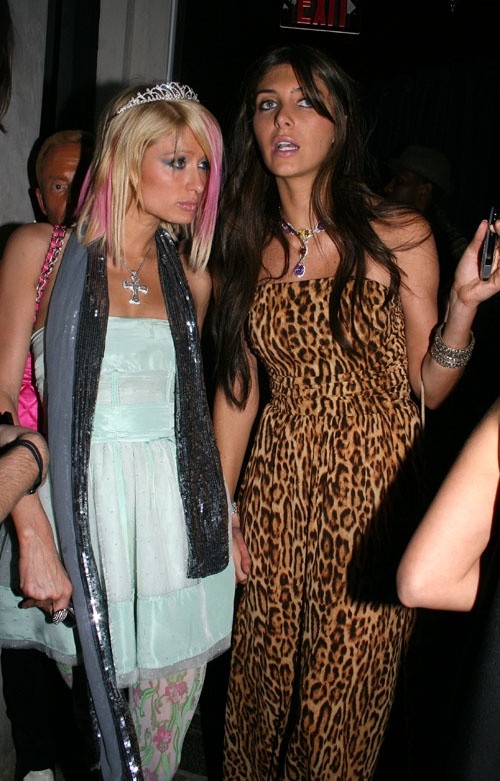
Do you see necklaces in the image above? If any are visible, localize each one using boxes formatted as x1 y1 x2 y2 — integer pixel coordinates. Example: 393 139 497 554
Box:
279 217 327 277
119 239 155 305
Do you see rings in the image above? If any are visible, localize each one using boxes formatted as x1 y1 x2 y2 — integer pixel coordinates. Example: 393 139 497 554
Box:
52 609 69 624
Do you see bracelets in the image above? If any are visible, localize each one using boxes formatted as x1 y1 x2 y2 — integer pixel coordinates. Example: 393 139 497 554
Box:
430 322 475 369
2 439 44 494
232 502 237 517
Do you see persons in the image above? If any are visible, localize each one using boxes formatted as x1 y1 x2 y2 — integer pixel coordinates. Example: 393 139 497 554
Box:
0 0 237 781
397 394 500 781
201 39 500 781
